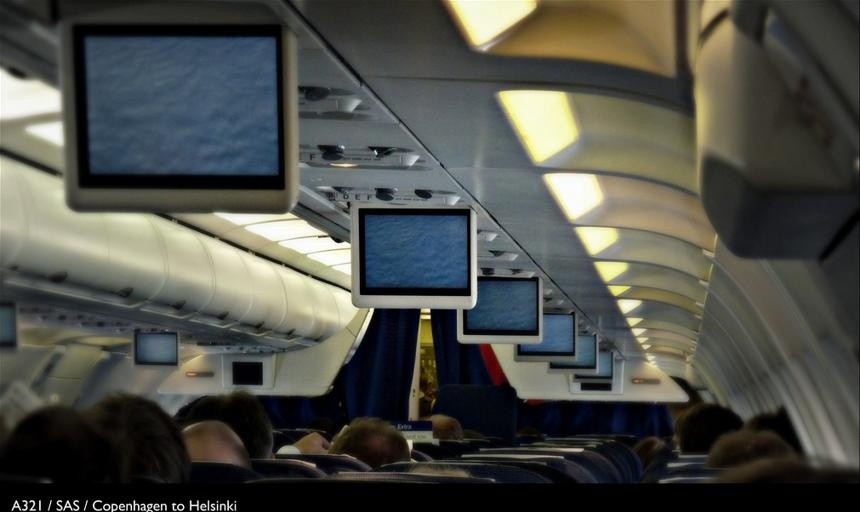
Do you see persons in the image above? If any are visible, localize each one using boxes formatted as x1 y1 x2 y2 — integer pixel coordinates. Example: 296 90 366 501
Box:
633 403 860 485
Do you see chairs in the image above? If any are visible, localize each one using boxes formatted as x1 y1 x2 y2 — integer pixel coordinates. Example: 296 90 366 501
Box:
1 429 859 511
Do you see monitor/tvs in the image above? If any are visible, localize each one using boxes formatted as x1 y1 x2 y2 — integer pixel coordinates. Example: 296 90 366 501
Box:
221 353 276 389
132 331 180 368
58 15 301 214
569 359 625 394
350 200 479 311
572 350 616 384
513 312 579 362
0 302 18 349
456 276 544 345
546 333 600 375
